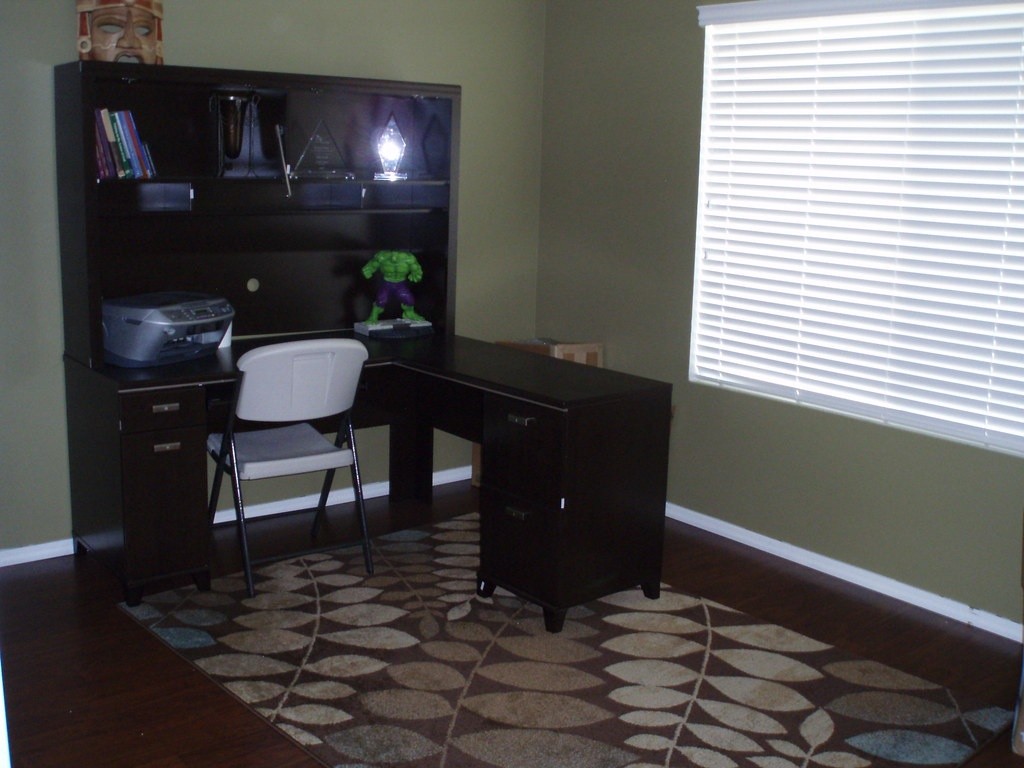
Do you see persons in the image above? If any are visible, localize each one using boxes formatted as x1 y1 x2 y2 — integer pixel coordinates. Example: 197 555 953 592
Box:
363 250 424 325
76 0 163 64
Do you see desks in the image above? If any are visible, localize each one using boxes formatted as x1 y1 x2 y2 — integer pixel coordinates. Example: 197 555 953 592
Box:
64 336 673 631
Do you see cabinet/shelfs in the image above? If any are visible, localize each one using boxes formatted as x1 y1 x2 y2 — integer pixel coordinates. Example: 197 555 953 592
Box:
55 60 461 366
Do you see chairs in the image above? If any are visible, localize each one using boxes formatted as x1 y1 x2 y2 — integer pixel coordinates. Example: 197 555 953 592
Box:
204 337 373 598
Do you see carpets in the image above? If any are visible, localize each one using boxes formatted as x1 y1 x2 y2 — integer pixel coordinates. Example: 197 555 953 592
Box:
115 512 1015 768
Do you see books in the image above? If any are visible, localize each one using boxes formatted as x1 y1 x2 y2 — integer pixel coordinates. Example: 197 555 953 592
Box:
95 107 156 179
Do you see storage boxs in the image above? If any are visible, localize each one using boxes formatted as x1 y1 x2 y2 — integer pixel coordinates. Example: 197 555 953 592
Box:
469 341 603 487
